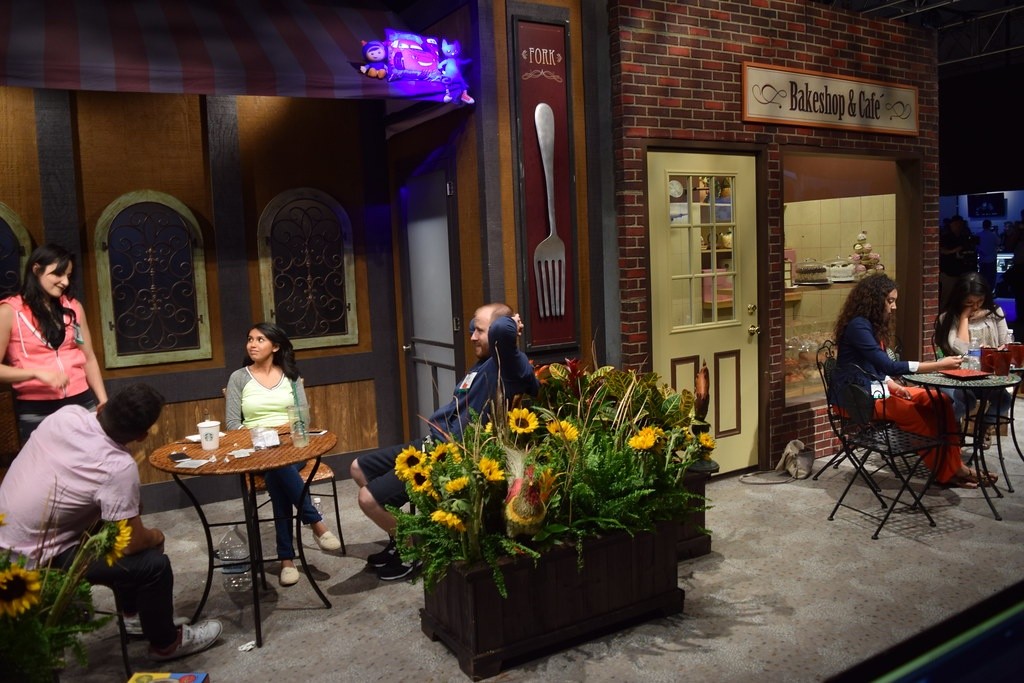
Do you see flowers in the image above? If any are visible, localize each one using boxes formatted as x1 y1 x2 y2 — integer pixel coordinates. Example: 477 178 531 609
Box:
382 390 717 597
0 510 131 683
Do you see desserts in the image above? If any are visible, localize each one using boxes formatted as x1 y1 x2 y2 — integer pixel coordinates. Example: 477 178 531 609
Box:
851 233 883 278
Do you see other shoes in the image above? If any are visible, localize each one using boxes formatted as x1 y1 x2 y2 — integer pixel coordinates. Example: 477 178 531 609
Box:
313 528 341 551
281 563 299 585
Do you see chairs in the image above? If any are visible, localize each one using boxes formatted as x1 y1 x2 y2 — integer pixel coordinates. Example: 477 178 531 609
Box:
810 312 979 540
1 450 136 679
223 388 347 568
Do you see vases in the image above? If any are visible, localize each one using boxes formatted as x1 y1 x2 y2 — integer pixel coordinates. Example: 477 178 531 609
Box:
420 516 686 683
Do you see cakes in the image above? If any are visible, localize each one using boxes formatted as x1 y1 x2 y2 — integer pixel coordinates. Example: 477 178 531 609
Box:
794 266 828 283
825 262 856 278
785 350 825 384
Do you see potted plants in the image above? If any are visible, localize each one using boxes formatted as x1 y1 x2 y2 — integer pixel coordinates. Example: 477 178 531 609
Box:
535 361 714 560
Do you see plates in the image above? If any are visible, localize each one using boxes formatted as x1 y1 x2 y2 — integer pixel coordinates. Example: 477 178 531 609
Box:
937 369 993 379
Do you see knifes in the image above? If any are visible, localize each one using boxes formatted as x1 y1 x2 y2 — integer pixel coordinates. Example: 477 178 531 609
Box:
532 102 568 317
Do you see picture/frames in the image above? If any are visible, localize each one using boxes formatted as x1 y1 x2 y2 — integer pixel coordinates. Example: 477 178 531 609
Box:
741 61 920 137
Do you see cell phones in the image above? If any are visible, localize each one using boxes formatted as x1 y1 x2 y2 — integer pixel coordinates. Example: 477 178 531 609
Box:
300 430 328 435
961 353 966 359
168 452 191 462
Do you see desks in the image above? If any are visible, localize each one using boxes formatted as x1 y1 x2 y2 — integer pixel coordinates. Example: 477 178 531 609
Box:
900 362 1024 520
148 426 338 648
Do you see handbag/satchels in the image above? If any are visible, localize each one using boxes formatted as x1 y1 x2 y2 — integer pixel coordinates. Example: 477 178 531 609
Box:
775 440 816 479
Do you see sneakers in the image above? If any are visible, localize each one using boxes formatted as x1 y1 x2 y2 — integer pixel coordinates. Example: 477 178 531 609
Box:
380 548 423 580
148 620 223 661
123 612 191 634
367 539 397 568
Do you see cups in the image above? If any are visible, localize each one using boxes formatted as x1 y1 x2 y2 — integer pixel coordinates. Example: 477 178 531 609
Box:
981 347 997 373
985 350 1012 376
285 405 309 448
197 419 221 451
1005 342 1024 368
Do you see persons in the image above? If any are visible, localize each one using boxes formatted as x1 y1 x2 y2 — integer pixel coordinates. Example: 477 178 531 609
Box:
833 274 999 489
939 270 1008 449
350 303 538 581
225 323 342 586
0 244 108 444
939 210 1024 310
0 383 223 662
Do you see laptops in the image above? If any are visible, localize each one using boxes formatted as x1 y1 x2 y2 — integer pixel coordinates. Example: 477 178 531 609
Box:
996 251 1014 275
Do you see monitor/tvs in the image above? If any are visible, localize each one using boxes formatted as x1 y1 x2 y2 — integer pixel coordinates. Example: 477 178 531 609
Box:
968 193 1004 218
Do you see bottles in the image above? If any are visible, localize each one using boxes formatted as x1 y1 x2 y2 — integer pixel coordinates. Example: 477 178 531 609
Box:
968 337 981 371
1004 329 1014 346
218 524 252 592
313 498 324 525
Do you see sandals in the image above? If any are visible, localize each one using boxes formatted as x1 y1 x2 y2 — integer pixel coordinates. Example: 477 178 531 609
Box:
982 433 992 450
934 478 976 489
966 467 998 485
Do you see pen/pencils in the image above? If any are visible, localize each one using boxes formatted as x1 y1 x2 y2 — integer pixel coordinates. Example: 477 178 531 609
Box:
278 431 290 436
175 442 201 445
